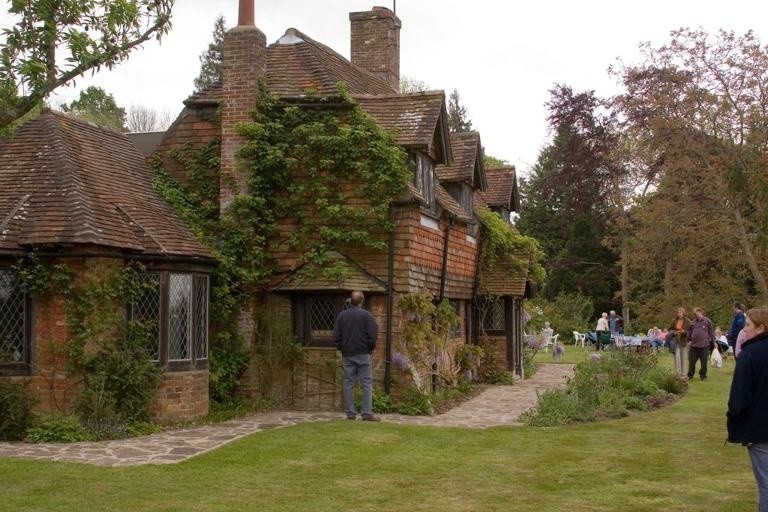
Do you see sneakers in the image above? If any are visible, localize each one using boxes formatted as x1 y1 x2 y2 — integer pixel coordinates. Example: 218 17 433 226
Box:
347 413 356 419
362 414 381 421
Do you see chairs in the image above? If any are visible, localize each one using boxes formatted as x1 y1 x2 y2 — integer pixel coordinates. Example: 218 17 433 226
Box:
572 330 586 348
546 332 560 347
614 333 651 356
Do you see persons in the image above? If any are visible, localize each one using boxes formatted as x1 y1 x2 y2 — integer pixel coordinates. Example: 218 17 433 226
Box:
727 306 768 512
541 321 551 331
595 310 623 350
632 301 748 380
332 289 382 421
